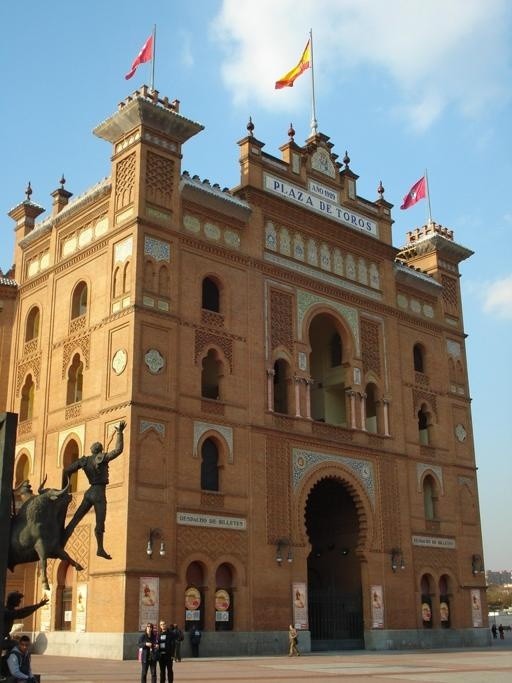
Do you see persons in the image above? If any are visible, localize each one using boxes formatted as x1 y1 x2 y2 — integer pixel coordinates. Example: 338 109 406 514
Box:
189 623 202 657
287 624 300 657
136 622 159 682
61 421 127 559
156 618 178 682
491 623 498 639
0 590 52 654
5 633 38 682
499 622 504 640
170 622 185 663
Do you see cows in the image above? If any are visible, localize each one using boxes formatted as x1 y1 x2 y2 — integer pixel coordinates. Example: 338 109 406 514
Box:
8 474 86 591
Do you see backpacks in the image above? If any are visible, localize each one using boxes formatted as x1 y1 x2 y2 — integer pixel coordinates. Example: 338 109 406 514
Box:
0 650 21 678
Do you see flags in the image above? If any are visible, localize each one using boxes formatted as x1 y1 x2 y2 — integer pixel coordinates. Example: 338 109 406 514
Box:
399 168 429 210
124 22 157 79
275 26 312 88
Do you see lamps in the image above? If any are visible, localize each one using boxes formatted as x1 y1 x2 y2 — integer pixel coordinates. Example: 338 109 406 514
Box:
473 553 485 576
276 536 292 565
391 548 405 573
147 527 165 559
